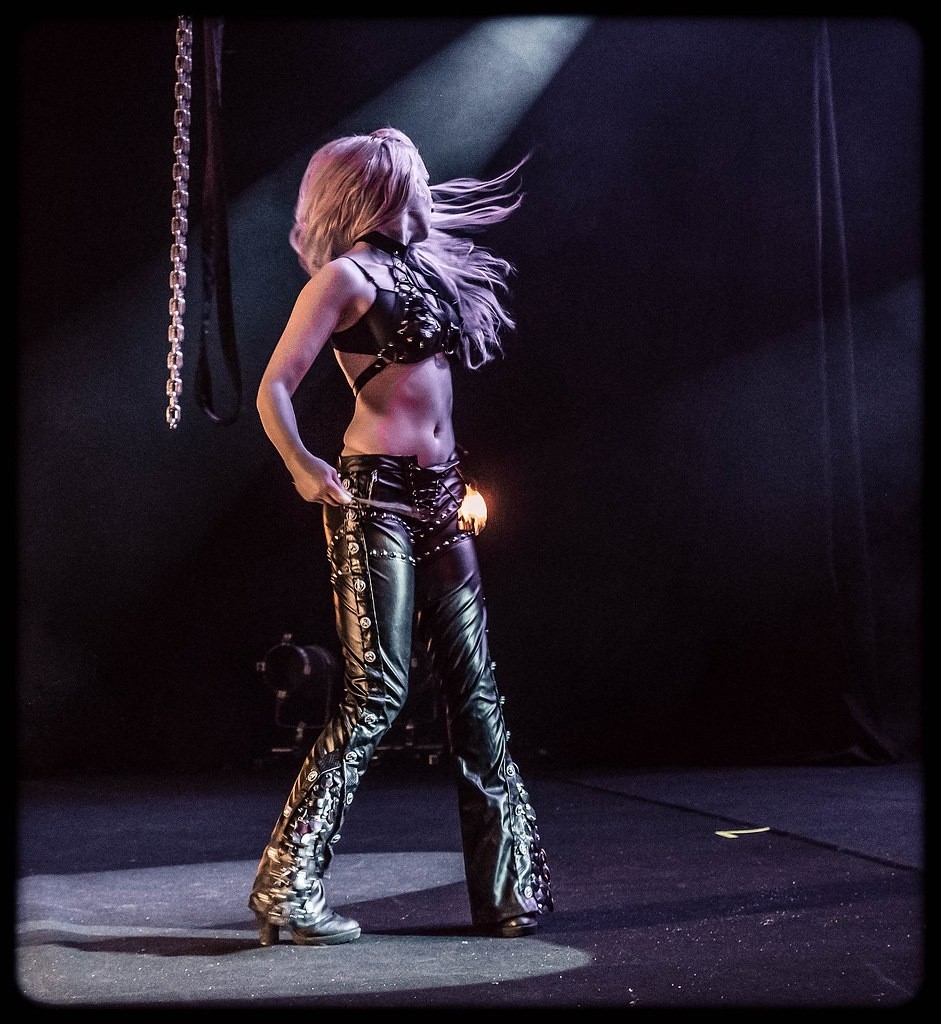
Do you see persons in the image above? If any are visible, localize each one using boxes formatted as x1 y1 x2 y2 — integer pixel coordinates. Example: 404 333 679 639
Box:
239 127 560 949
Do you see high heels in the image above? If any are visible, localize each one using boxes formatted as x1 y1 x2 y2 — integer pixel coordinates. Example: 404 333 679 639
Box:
253 907 362 946
494 915 540 936
